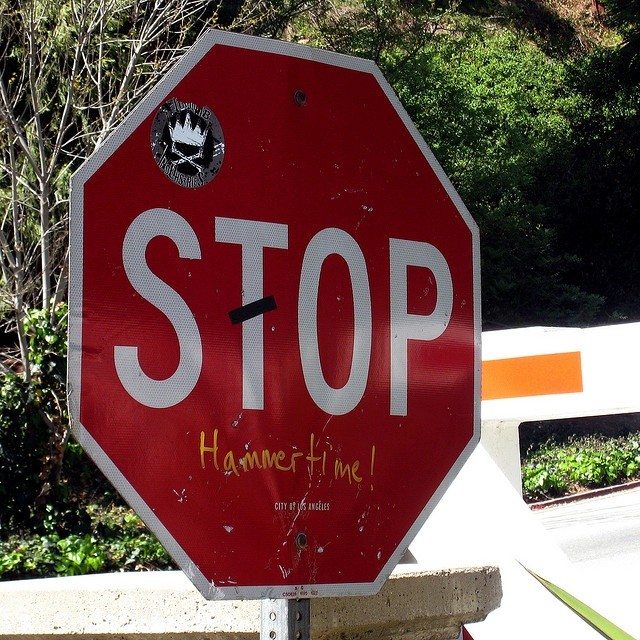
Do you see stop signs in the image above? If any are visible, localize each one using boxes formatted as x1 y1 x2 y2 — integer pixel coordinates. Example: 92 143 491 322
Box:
68 29 481 599
